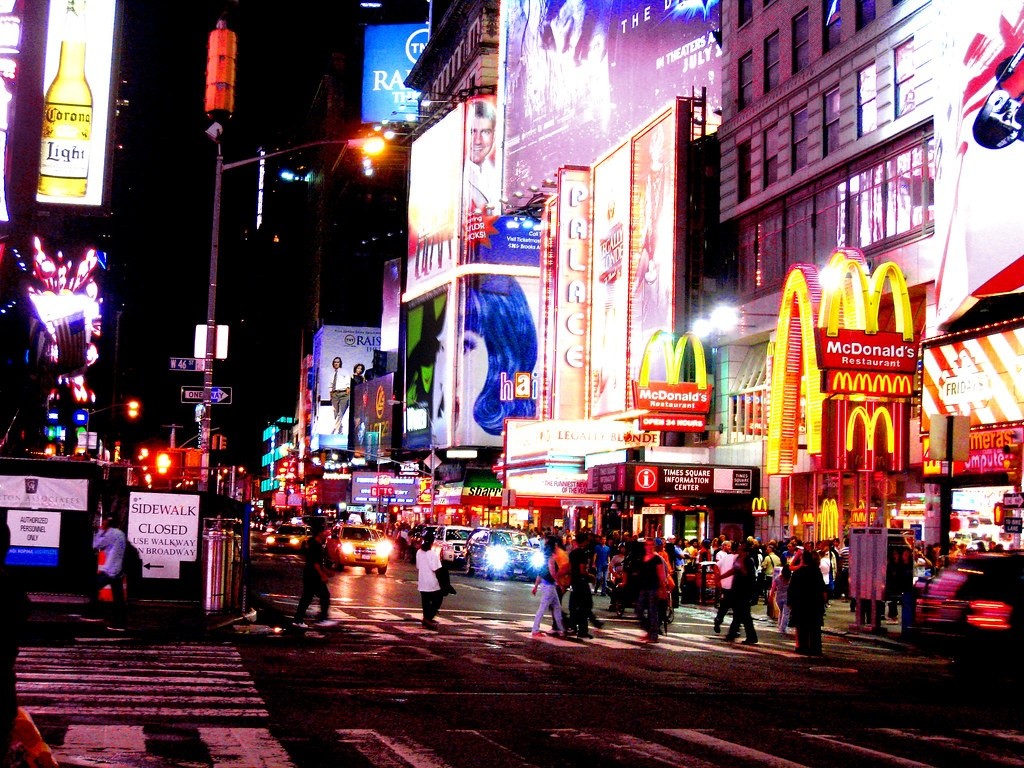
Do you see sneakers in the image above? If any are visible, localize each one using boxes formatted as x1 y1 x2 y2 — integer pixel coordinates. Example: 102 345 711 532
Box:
532 632 546 639
314 619 333 626
293 620 308 628
560 628 574 636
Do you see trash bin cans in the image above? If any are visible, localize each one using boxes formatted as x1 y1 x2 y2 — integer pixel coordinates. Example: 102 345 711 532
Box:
200 513 242 618
696 561 718 606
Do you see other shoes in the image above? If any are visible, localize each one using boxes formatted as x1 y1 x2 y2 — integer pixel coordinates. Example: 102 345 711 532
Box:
547 629 560 635
742 635 759 644
724 634 736 642
576 632 594 640
713 617 722 634
421 616 437 628
645 637 658 644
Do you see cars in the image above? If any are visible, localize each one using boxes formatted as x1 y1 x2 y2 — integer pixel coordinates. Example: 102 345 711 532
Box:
324 524 392 576
406 524 476 572
464 527 546 582
913 552 1023 667
266 524 311 550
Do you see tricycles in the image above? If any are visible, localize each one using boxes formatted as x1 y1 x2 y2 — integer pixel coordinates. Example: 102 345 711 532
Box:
613 541 682 625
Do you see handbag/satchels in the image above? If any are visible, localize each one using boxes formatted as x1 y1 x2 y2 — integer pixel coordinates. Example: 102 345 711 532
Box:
767 591 779 622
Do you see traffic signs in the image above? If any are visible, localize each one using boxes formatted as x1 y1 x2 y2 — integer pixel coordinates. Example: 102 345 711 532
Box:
180 386 232 404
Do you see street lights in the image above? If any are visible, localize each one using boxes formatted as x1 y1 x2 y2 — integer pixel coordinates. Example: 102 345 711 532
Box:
196 136 388 492
85 401 139 452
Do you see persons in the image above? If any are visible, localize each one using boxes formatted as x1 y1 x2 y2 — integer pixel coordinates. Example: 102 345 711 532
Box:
850 548 912 624
292 517 334 628
330 356 365 434
480 523 594 550
364 518 370 526
913 539 1014 580
392 519 419 559
465 99 497 217
80 513 127 632
0 521 30 768
435 275 537 446
416 531 443 627
531 530 848 652
